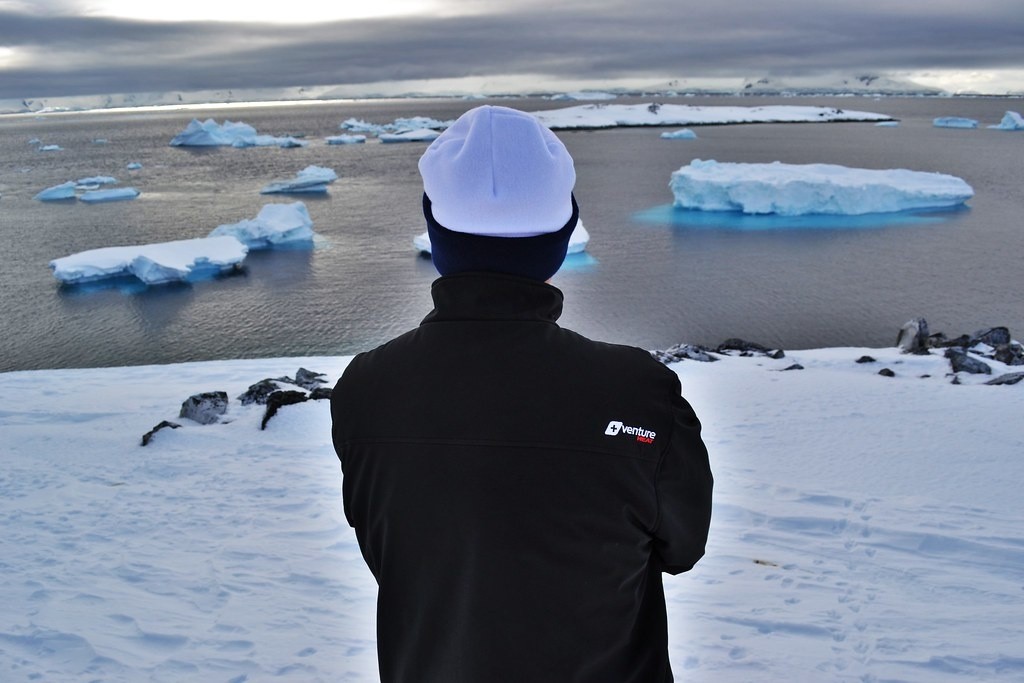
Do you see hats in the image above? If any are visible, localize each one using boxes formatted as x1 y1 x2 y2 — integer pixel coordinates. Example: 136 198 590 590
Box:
417 105 580 283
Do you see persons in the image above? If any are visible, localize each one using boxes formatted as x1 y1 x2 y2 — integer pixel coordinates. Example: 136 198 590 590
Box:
331 107 713 683
